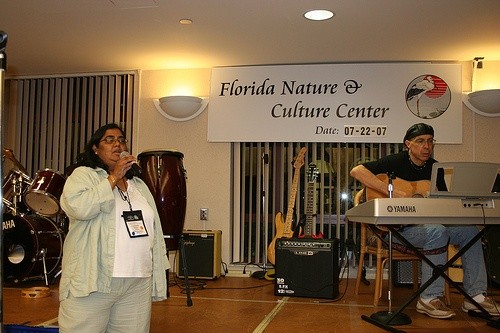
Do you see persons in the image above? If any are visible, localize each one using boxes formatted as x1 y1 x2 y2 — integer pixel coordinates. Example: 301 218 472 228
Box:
58 123 170 333
352 124 500 318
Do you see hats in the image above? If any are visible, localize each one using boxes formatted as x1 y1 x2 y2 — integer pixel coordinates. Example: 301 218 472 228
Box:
403 123 434 147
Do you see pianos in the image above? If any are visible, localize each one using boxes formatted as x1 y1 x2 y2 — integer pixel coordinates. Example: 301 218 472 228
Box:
344 194 500 333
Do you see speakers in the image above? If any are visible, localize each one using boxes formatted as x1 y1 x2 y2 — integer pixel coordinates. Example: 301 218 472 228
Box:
275 238 340 299
176 230 223 280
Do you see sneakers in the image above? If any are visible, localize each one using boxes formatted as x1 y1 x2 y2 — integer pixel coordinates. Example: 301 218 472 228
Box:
416 297 455 319
460 295 499 316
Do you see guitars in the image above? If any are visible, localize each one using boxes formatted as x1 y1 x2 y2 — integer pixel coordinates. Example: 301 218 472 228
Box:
363 175 433 202
267 147 324 268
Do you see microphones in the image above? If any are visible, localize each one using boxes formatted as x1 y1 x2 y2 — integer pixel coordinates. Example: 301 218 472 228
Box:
119 151 142 175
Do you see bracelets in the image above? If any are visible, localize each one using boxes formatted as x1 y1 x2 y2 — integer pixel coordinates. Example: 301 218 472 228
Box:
109 175 118 186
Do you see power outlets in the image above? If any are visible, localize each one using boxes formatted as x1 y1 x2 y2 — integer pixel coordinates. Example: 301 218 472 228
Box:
200 209 208 220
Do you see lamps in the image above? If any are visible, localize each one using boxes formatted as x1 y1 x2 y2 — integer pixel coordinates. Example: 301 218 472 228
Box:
153 96 210 122
462 88 500 117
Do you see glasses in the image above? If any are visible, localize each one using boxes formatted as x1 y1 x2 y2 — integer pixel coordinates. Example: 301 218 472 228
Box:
411 139 437 145
99 137 129 145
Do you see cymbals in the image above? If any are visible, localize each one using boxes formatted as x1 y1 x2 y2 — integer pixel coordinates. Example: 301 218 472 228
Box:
21 287 53 299
2 146 27 174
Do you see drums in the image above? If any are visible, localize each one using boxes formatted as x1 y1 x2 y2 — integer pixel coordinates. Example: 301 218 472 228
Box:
0 167 67 288
136 150 186 252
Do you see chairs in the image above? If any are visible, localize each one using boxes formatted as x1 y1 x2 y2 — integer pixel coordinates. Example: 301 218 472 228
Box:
354 189 451 308
